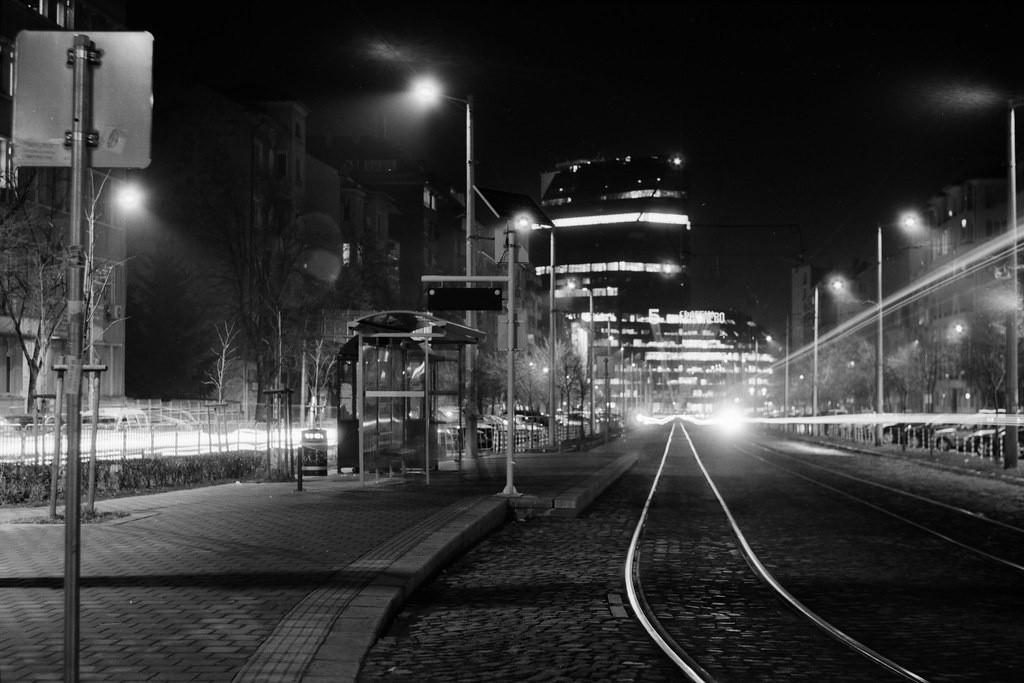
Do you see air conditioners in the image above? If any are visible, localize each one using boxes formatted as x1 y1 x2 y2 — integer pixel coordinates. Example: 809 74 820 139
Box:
109 305 122 320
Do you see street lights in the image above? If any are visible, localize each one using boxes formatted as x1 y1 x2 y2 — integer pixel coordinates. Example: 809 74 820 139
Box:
414 82 479 459
568 280 595 437
754 331 771 418
876 215 915 445
608 317 638 434
706 337 758 418
813 281 842 436
518 217 556 444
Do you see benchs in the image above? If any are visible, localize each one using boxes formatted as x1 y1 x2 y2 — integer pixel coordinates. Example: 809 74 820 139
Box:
374 447 416 484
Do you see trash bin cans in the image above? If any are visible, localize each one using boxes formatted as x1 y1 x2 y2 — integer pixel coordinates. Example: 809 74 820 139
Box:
301 429 327 476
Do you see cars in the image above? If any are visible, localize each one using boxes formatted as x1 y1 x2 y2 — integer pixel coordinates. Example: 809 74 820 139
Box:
883 410 1024 457
